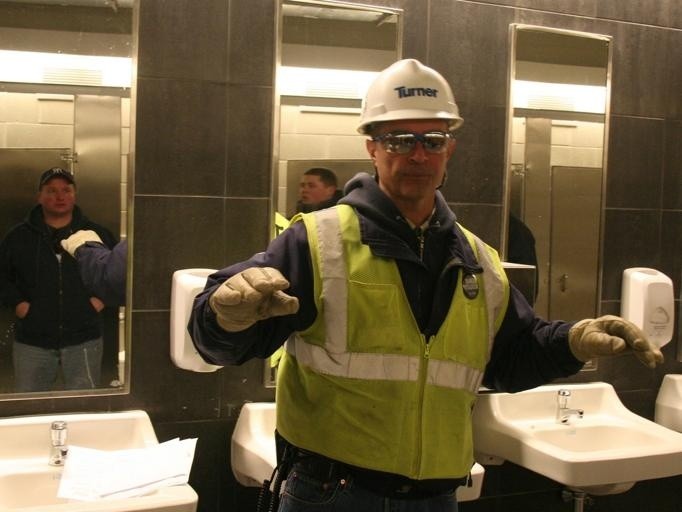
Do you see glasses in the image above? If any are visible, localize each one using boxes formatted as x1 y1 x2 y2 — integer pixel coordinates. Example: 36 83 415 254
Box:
374 130 451 154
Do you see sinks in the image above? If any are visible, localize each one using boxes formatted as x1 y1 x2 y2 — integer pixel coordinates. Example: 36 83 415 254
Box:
470 380 682 488
0 409 197 512
230 402 484 503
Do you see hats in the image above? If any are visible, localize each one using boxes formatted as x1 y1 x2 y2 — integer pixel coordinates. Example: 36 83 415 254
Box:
39 166 73 184
356 58 464 134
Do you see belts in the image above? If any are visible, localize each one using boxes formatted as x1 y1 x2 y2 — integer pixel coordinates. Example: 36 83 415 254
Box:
292 450 466 499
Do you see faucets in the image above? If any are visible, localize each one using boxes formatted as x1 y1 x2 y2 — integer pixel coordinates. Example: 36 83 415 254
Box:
556 389 585 424
47 422 69 465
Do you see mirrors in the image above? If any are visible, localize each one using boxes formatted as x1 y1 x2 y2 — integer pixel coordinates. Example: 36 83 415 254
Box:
268 0 404 248
0 0 139 402
500 22 615 373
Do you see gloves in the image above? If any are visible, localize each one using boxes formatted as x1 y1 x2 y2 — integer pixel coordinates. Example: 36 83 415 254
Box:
60 229 104 259
568 315 664 369
209 267 299 332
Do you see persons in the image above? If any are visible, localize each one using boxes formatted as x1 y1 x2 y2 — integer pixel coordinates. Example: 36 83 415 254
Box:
2 168 121 393
185 57 668 510
60 229 128 309
295 165 344 216
504 209 539 314
